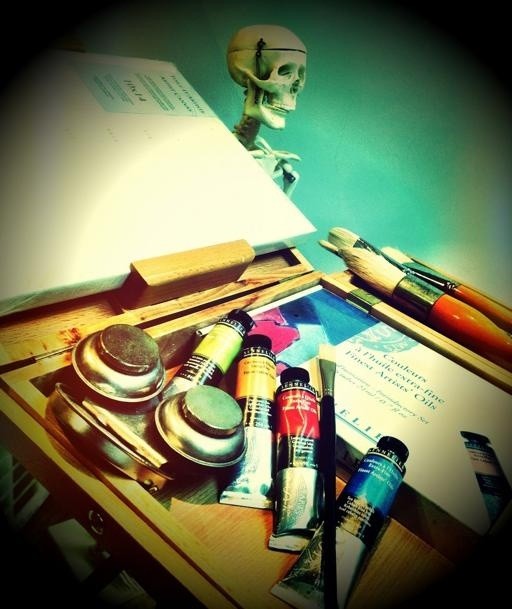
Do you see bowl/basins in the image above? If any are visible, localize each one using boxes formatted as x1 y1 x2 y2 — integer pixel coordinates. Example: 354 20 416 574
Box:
70 322 166 404
152 382 250 470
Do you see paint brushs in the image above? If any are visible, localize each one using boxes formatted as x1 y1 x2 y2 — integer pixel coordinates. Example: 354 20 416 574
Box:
319 358 337 609
318 226 512 374
